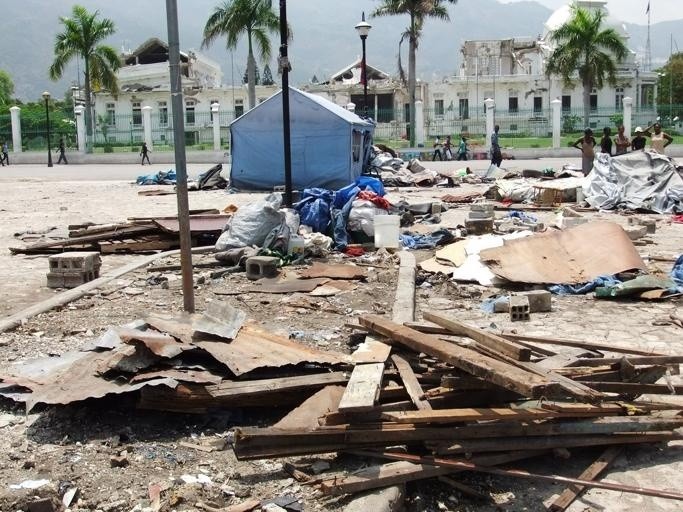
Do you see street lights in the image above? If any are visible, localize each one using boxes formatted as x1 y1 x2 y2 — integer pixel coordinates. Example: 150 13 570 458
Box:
658 72 665 123
355 12 371 119
42 92 53 167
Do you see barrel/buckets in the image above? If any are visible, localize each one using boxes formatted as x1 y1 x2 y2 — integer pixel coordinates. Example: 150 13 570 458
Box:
373 214 401 249
287 234 305 256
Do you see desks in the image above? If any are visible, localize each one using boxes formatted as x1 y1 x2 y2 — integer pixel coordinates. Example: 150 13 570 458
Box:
532 176 583 207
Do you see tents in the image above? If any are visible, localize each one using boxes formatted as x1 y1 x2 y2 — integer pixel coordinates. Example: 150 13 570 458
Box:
229 85 377 195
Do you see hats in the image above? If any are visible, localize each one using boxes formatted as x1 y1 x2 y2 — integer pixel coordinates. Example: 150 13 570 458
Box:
634 127 643 133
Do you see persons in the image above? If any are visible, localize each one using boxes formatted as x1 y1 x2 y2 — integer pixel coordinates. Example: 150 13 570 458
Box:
642 122 674 155
139 140 151 164
613 125 631 155
490 124 503 166
631 127 648 150
455 136 469 160
600 127 613 154
431 136 442 161
443 133 454 160
0 140 10 165
55 138 68 165
573 127 595 177
